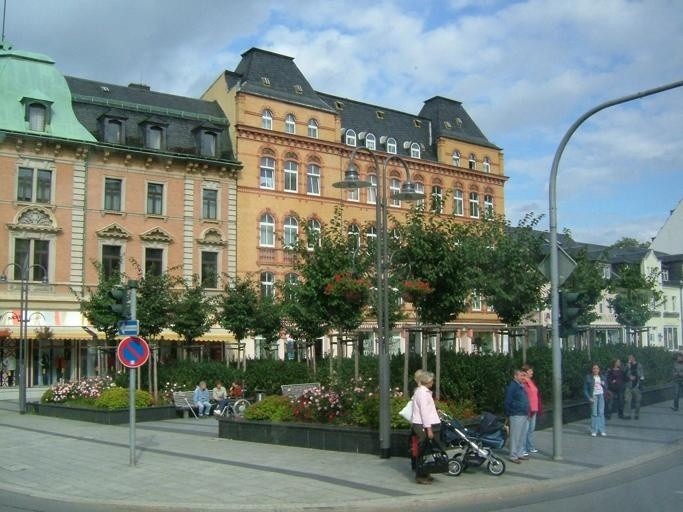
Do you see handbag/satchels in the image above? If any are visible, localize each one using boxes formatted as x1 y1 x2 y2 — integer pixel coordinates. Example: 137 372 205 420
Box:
416 438 449 475
605 390 613 400
399 397 413 422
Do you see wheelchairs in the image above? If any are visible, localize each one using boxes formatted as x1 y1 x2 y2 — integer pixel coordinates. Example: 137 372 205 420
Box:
215 385 250 420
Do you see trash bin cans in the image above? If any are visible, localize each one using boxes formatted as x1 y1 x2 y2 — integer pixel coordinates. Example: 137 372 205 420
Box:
254 390 266 403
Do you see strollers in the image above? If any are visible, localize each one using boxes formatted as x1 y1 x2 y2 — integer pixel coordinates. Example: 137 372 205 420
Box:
437 408 509 476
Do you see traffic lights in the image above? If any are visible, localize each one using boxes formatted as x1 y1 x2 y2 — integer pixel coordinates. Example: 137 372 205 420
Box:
107 285 128 320
556 293 584 337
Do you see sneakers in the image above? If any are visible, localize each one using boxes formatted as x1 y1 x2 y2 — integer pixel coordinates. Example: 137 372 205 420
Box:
600 432 607 436
591 432 597 436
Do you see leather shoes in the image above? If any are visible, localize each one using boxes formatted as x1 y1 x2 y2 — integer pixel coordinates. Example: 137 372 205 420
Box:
416 480 432 484
510 450 538 464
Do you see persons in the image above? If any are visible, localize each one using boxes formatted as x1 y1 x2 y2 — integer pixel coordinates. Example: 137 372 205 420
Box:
622 352 645 421
212 379 243 416
668 349 682 412
210 380 227 416
582 363 606 437
192 380 211 419
410 369 426 474
502 369 530 465
38 354 49 386
605 358 635 421
520 365 538 457
410 371 443 486
54 353 65 382
3 351 16 388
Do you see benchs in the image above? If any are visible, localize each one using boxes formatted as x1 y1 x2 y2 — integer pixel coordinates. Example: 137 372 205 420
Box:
173 390 246 418
280 383 322 396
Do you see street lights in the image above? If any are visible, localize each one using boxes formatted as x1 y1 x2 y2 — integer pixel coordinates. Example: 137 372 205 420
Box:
332 146 426 460
0 263 48 416
